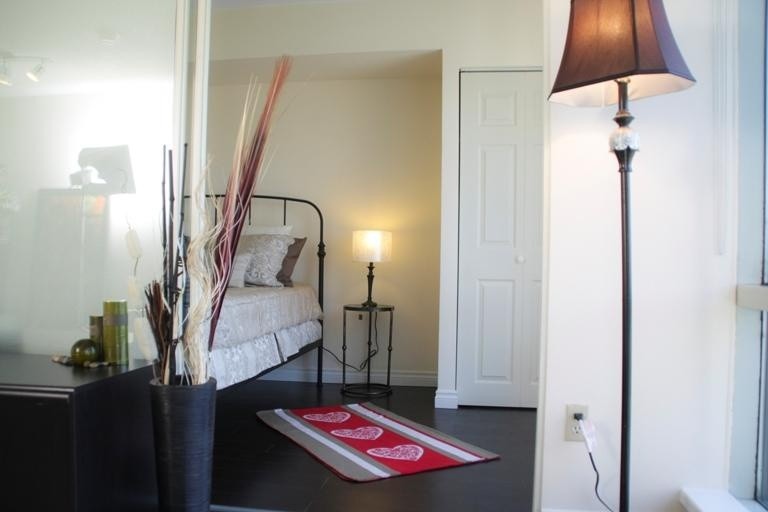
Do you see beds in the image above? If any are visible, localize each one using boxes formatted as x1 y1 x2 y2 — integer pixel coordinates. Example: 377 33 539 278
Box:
180 191 326 390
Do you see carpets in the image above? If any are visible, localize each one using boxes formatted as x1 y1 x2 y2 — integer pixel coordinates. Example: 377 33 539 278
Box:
255 399 502 483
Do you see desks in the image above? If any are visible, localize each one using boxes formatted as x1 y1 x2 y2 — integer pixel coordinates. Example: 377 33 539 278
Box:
340 303 395 399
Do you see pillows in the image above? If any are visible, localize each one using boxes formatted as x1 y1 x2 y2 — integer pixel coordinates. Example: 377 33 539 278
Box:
239 223 292 237
234 235 295 288
275 237 306 288
226 252 248 289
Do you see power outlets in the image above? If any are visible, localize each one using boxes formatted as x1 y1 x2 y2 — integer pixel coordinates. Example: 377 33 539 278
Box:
565 406 600 443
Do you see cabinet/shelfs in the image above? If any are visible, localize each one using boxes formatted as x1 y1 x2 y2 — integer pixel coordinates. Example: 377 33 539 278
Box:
1 349 163 510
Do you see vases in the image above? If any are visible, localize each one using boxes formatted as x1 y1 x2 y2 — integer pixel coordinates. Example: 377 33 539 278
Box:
147 373 217 511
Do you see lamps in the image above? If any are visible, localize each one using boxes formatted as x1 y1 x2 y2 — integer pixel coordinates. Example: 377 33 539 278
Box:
351 229 393 307
547 1 697 512
